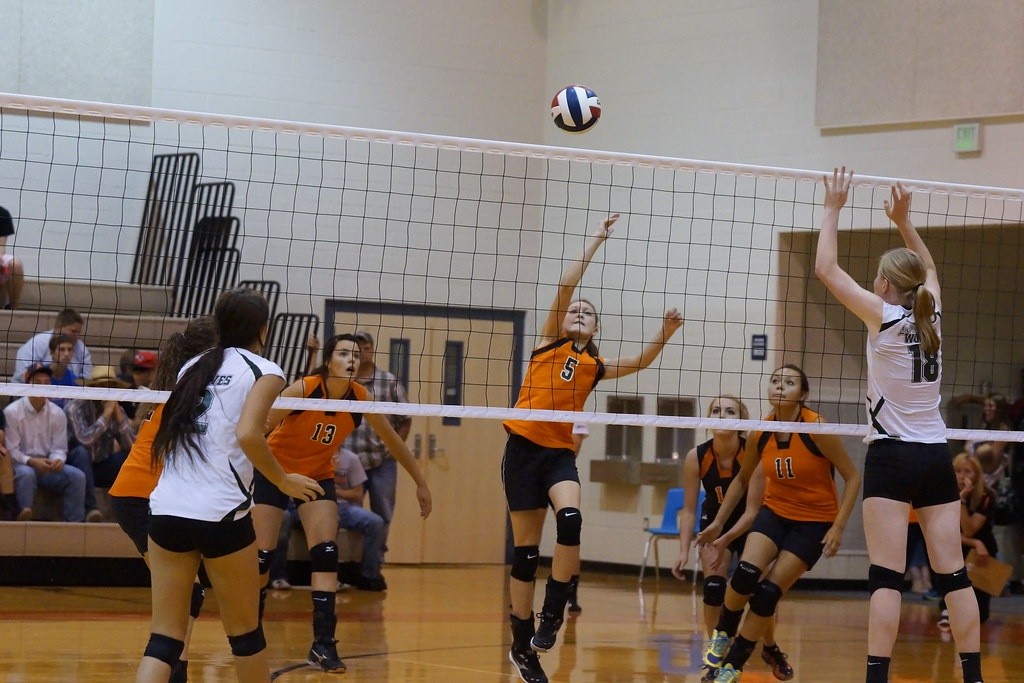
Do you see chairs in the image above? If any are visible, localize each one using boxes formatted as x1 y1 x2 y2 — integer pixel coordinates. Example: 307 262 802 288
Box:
639 486 707 586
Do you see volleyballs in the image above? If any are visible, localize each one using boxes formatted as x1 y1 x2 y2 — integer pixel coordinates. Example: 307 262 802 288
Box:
551 85 602 134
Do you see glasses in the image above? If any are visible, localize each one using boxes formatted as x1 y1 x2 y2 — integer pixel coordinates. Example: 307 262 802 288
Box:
133 367 149 372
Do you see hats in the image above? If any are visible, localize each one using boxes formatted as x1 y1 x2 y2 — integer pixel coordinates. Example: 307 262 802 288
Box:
355 331 372 344
75 366 132 389
24 363 52 384
133 352 158 369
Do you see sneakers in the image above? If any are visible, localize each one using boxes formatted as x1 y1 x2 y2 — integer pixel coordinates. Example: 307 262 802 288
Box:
762 643 794 681
703 629 734 668
307 640 346 673
530 607 564 653
509 642 548 683
713 663 742 683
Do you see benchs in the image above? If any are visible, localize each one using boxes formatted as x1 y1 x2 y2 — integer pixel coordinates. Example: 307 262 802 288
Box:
0 278 365 561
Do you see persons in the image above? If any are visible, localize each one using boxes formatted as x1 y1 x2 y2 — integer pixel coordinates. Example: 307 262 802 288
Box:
117 348 138 385
7 308 92 403
906 392 1024 630
272 510 291 589
43 336 83 409
119 351 156 420
814 166 987 682
135 288 272 683
108 315 271 683
307 330 413 552
3 367 102 522
672 365 861 683
249 333 432 673
500 213 684 683
0 205 24 310
290 447 388 591
62 366 137 522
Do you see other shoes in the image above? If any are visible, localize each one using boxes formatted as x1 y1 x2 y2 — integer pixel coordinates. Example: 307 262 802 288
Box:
936 608 950 625
271 579 290 590
569 596 581 612
85 509 103 522
352 569 388 591
17 507 32 520
